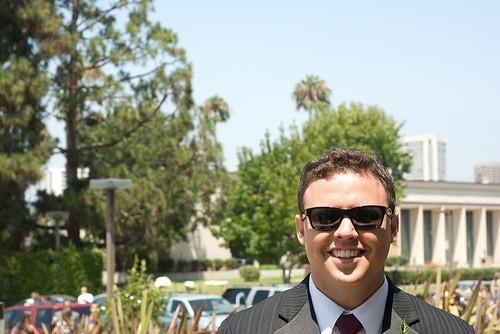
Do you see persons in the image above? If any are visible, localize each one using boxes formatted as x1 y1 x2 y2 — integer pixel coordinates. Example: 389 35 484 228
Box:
10 286 102 334
477 272 500 306
214 146 476 334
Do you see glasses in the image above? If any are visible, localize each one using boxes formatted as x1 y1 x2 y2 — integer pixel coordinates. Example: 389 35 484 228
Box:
305 205 393 231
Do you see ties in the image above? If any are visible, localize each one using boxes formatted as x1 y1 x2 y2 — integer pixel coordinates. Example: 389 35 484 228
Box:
335 313 364 334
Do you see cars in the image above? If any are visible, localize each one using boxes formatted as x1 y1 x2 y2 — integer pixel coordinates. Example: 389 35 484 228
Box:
456 278 493 296
5 303 92 334
91 291 178 329
220 283 300 308
158 293 248 333
